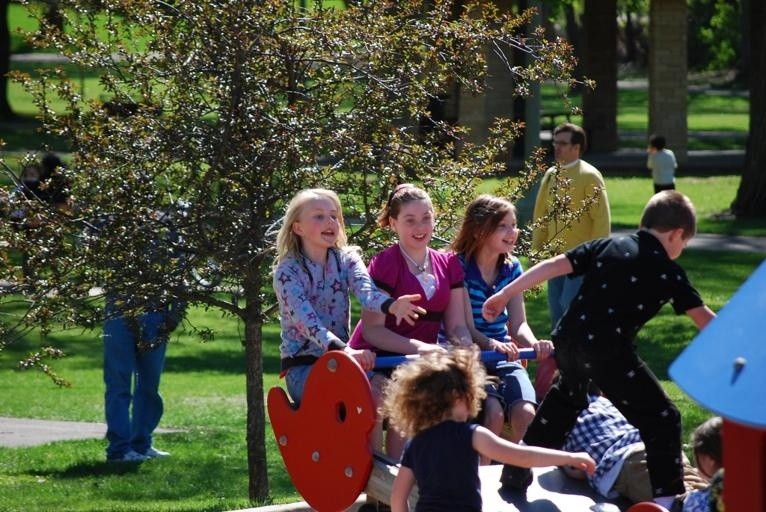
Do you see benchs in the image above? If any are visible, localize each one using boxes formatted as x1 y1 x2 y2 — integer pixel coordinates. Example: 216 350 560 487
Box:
541 110 582 132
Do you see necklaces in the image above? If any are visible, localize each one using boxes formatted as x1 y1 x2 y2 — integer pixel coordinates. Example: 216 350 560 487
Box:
395 242 430 287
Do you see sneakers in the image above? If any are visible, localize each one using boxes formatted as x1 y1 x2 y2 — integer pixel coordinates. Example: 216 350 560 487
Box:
503 471 533 488
107 450 151 461
145 445 171 456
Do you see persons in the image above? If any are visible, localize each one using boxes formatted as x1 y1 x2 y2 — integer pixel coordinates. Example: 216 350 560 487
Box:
530 122 613 332
437 198 554 467
270 187 410 511
381 345 597 512
648 133 677 192
680 416 723 511
482 191 718 512
83 177 188 465
346 183 477 379
14 149 77 222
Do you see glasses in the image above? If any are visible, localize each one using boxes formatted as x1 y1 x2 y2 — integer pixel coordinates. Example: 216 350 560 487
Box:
552 138 570 146
